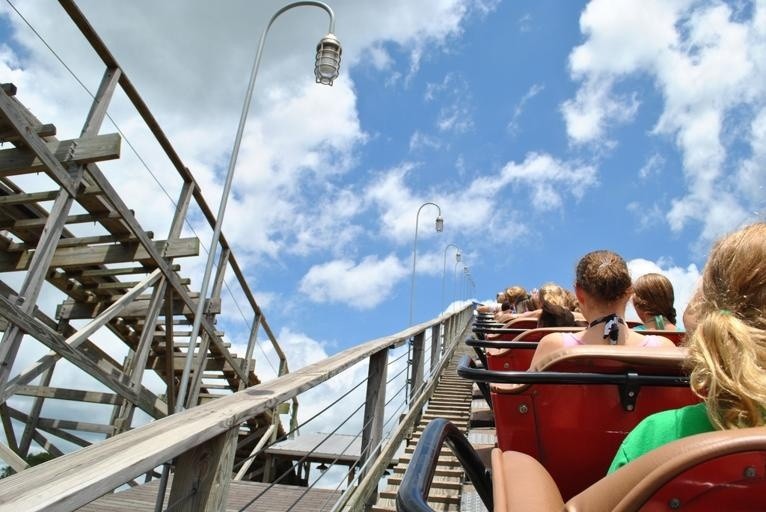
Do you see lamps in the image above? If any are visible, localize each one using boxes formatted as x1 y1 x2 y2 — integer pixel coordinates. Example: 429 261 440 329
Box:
316 463 328 473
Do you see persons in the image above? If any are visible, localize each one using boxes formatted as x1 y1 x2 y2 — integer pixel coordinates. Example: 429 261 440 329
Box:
632 273 682 331
604 221 766 475
530 250 679 367
478 282 582 327
